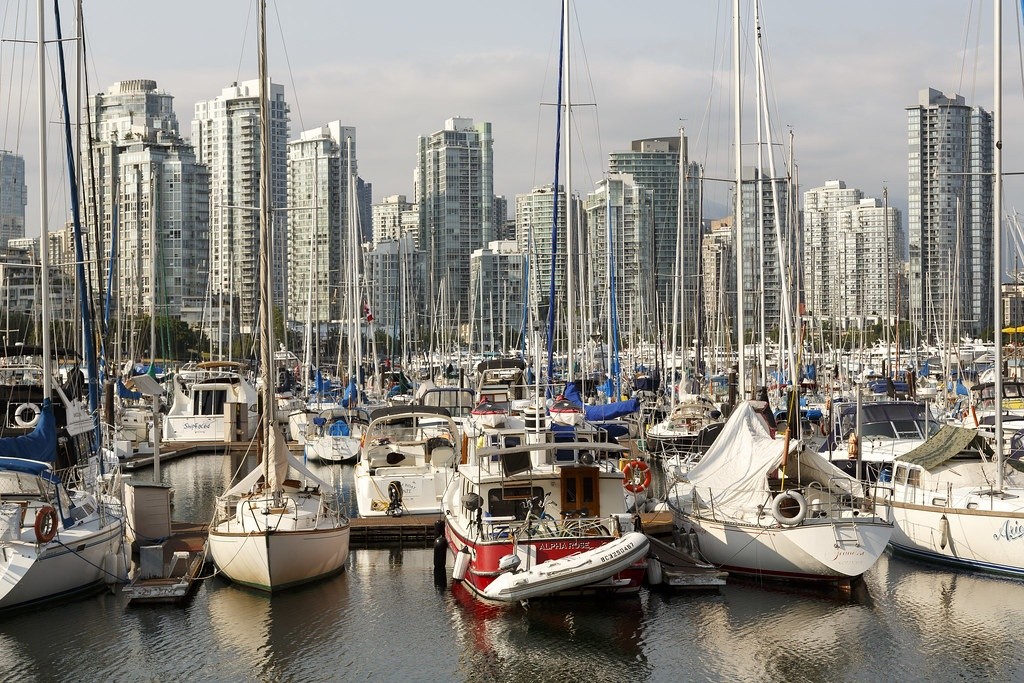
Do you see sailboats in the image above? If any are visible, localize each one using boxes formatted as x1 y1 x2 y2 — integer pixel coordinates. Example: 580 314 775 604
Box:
0 0 1024 615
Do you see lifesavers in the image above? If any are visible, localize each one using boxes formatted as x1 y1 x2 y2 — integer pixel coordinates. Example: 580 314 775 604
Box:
35 506 58 543
15 403 41 427
623 462 651 494
771 490 807 525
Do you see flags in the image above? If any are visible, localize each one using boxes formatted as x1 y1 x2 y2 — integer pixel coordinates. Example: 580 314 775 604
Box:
364 300 373 322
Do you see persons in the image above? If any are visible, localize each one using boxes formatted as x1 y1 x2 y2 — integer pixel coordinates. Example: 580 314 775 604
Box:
67 363 84 402
